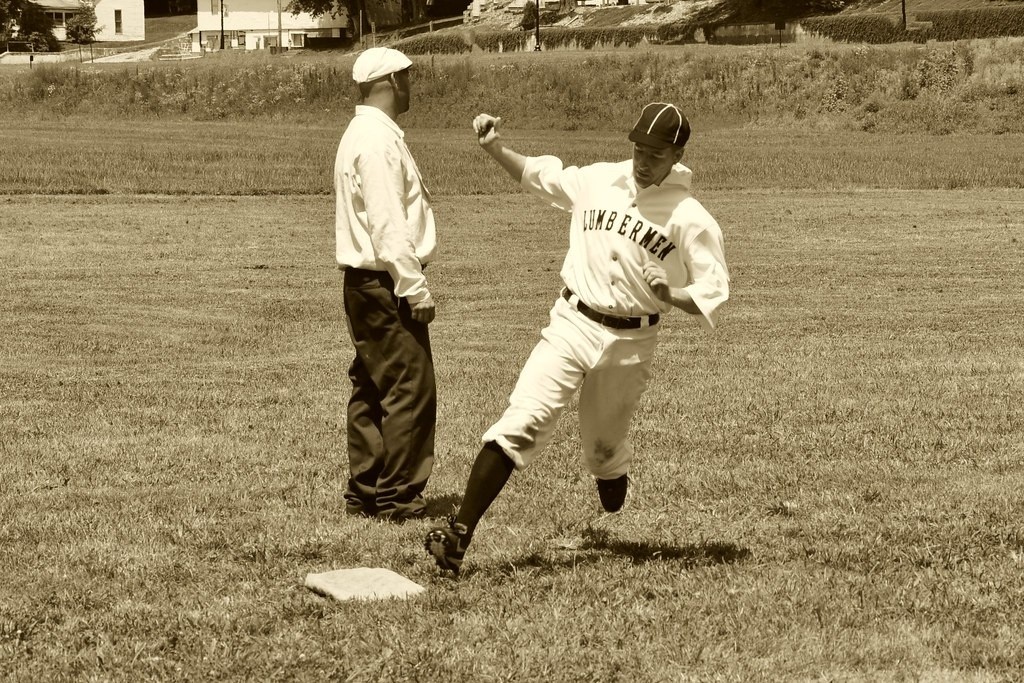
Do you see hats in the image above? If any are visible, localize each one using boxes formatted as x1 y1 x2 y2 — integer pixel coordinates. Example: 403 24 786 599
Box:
627 102 692 150
351 45 413 84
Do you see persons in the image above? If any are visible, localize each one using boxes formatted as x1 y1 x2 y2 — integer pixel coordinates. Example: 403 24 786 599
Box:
335 47 439 522
424 102 731 569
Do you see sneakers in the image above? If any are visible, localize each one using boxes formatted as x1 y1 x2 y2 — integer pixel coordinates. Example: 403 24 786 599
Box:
425 513 466 571
595 473 631 513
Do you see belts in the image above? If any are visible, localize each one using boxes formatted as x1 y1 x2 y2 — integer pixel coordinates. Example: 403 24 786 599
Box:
563 290 660 329
